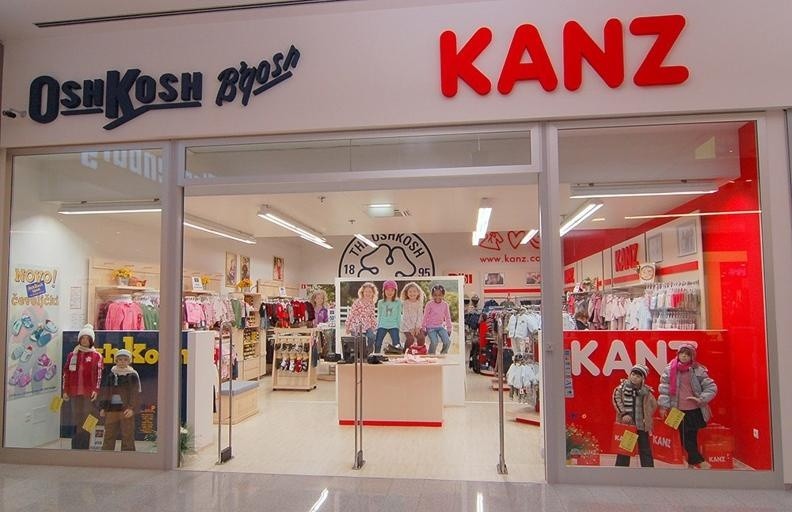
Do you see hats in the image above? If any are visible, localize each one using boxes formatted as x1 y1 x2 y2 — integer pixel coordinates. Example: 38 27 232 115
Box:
114 348 133 366
383 279 398 291
677 342 696 362
630 362 649 379
77 322 96 345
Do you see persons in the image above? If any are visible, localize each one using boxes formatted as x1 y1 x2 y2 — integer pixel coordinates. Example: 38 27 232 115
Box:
475 313 490 367
310 290 328 327
422 284 452 354
612 363 658 467
374 280 402 353
61 323 103 449
98 349 142 450
657 343 718 472
399 282 425 356
345 282 378 352
575 312 595 331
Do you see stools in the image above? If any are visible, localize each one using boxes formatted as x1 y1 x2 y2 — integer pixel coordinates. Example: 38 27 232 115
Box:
213 380 260 427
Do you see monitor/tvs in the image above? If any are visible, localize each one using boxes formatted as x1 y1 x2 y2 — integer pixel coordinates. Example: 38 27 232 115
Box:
342 337 365 363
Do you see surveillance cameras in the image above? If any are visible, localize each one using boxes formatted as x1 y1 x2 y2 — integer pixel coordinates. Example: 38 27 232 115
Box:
1 107 26 119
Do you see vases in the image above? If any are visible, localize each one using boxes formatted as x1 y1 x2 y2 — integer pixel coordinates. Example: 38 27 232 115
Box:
116 276 131 289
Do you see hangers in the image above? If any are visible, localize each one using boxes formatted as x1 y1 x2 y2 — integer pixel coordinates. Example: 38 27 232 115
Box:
262 296 311 305
506 304 544 314
101 291 240 304
643 279 703 326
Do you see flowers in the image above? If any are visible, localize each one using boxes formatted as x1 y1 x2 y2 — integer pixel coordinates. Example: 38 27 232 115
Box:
236 278 253 289
200 276 210 284
109 265 134 279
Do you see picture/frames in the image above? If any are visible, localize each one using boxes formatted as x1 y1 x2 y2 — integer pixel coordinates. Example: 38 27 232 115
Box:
675 218 700 258
646 232 664 266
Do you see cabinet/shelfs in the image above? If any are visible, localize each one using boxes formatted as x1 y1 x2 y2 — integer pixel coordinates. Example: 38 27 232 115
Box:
272 327 319 392
230 290 263 382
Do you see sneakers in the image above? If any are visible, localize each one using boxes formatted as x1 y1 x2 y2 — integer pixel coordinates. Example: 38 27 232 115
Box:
37 333 51 348
37 352 52 366
8 367 24 386
30 326 43 342
700 461 712 470
21 314 33 329
19 374 32 387
19 344 33 363
11 345 25 360
11 319 22 337
45 361 57 380
43 319 59 333
33 367 47 381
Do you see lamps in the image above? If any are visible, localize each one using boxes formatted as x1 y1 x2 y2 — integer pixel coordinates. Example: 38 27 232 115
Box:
349 220 378 250
570 179 719 201
56 198 163 216
182 211 257 244
475 196 495 240
256 204 326 243
520 225 540 245
560 198 606 237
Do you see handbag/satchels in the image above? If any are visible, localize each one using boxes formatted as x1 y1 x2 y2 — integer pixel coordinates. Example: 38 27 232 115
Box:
611 421 639 457
647 412 687 465
696 422 736 471
